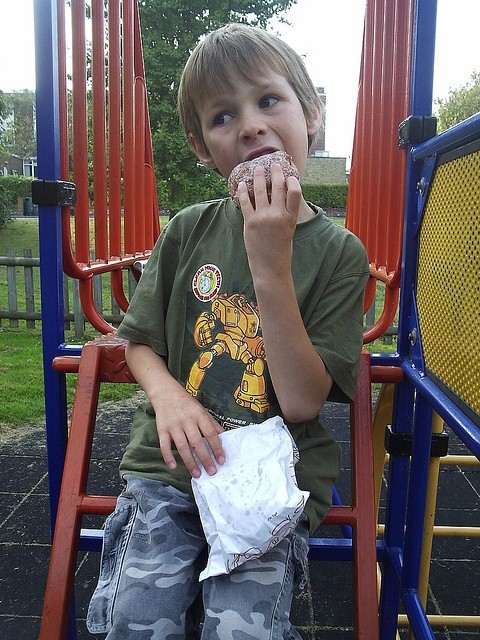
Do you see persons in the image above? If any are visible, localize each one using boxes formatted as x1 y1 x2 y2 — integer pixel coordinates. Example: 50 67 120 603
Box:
86 22 369 639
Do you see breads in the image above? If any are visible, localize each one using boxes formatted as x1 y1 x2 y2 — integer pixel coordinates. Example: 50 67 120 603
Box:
227 150 301 212
